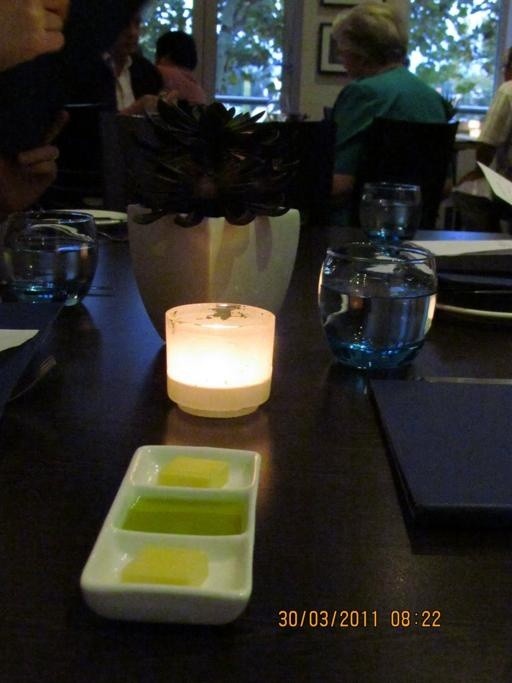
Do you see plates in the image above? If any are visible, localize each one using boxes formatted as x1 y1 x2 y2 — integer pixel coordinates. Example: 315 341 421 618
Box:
74 438 262 626
53 207 130 227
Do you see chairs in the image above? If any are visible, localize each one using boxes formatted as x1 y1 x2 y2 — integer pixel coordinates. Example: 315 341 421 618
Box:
253 107 460 228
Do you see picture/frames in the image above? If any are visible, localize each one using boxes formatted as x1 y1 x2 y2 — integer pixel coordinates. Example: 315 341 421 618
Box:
316 22 348 76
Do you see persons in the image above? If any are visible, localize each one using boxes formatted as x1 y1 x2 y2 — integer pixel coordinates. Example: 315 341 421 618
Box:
327 1 457 230
453 46 512 234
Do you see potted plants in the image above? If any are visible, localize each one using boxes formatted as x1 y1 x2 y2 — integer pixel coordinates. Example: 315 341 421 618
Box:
123 94 303 340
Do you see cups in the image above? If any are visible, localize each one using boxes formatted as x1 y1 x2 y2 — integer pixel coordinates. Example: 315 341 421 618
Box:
359 181 421 245
0 209 99 307
317 240 440 373
